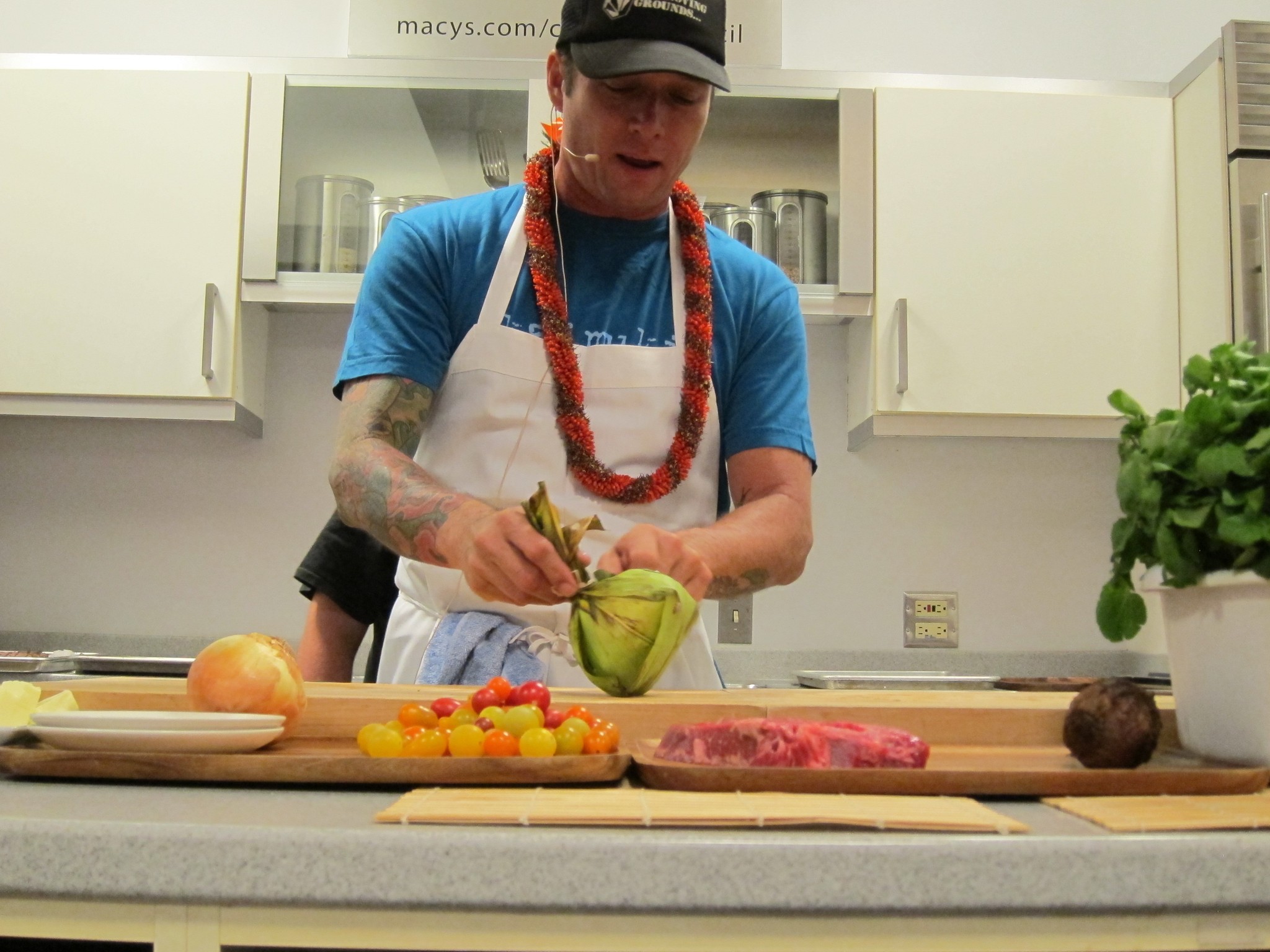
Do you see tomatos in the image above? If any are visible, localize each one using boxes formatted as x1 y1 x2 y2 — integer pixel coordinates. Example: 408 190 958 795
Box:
358 678 620 759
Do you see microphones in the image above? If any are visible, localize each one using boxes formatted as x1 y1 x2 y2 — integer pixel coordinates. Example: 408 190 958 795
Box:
554 108 601 163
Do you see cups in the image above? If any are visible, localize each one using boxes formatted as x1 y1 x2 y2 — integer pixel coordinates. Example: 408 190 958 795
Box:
750 188 828 285
292 175 374 273
400 195 450 206
359 196 422 273
710 206 776 264
702 202 739 222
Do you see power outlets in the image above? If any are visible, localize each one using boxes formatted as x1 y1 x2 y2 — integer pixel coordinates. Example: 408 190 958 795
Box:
904 592 959 649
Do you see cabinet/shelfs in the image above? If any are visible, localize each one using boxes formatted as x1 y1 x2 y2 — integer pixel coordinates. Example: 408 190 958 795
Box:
242 72 873 325
0 67 270 438
845 87 1182 449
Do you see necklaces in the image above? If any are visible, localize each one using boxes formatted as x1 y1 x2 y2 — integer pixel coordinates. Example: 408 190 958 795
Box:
524 144 714 502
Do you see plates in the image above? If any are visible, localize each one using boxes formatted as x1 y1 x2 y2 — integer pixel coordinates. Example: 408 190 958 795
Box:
0 727 30 744
28 711 286 753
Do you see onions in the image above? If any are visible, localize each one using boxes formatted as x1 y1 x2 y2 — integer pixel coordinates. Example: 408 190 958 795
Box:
187 634 309 749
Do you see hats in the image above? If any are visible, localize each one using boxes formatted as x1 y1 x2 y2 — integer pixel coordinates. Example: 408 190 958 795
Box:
556 0 734 94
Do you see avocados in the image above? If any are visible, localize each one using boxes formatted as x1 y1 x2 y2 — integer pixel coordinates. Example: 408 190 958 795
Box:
1060 676 1162 769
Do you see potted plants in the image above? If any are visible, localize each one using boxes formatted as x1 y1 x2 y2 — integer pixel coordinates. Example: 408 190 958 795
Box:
1094 340 1268 765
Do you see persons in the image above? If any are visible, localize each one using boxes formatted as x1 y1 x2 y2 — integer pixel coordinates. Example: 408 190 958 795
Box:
293 511 400 683
330 0 820 687
